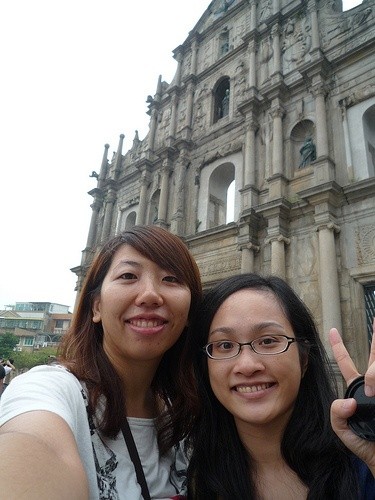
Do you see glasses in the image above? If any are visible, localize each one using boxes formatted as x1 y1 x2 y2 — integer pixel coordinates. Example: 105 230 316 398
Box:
203 334 306 360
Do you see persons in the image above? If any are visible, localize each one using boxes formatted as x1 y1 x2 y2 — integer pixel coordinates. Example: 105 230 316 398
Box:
299 136 315 167
0 225 205 500
0 357 16 398
48 354 66 364
187 273 375 500
153 207 158 223
222 89 229 117
221 40 228 54
